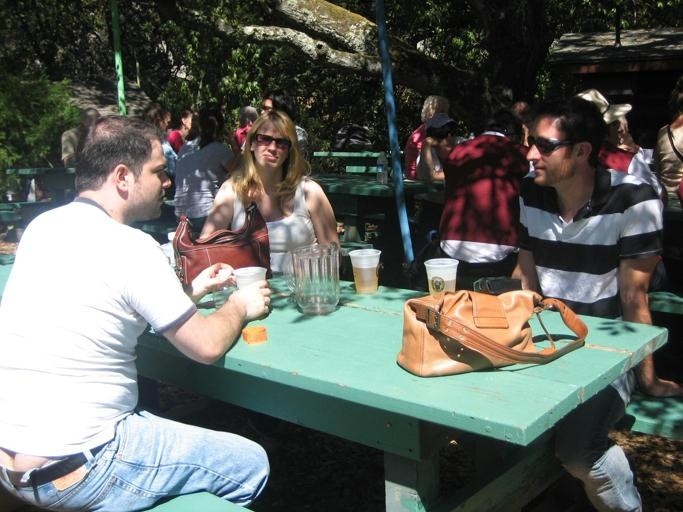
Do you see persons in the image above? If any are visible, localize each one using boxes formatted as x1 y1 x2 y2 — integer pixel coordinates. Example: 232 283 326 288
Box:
0 115 273 512
397 95 533 292
462 96 683 511
60 108 98 196
191 111 349 284
581 77 683 293
140 94 324 237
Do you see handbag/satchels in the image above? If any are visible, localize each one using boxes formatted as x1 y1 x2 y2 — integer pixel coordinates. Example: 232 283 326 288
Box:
174 209 272 294
397 274 543 377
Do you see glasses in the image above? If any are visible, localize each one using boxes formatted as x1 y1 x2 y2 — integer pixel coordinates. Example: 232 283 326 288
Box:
259 104 273 111
433 127 455 140
254 132 291 149
527 134 580 156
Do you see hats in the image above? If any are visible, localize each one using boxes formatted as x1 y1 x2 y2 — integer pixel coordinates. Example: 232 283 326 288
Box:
426 112 459 129
576 89 633 126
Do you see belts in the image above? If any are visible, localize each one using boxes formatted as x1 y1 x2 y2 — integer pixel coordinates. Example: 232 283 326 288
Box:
0 441 110 488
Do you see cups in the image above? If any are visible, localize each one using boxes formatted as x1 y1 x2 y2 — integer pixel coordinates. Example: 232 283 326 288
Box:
231 265 267 290
424 257 459 295
348 247 382 294
284 241 340 315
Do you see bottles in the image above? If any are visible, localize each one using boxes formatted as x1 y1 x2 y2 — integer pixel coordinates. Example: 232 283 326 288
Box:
376 151 388 185
